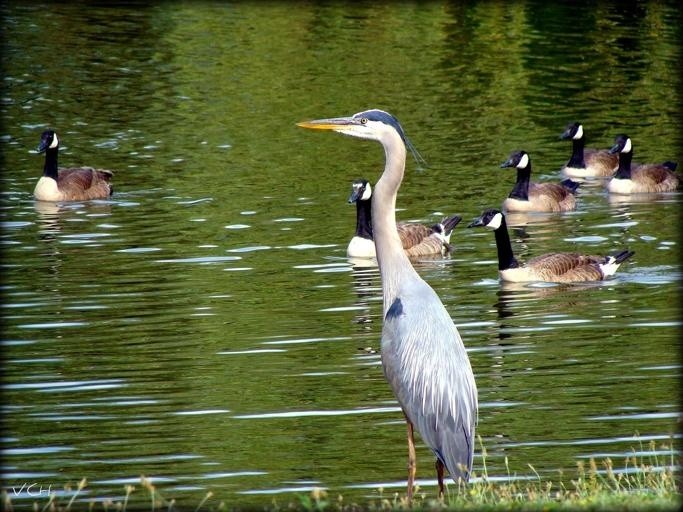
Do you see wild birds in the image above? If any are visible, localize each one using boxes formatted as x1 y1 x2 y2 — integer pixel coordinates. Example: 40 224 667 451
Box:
296 108 482 512
559 122 619 178
499 150 581 214
606 133 683 193
33 130 115 203
346 180 463 259
467 209 636 284
